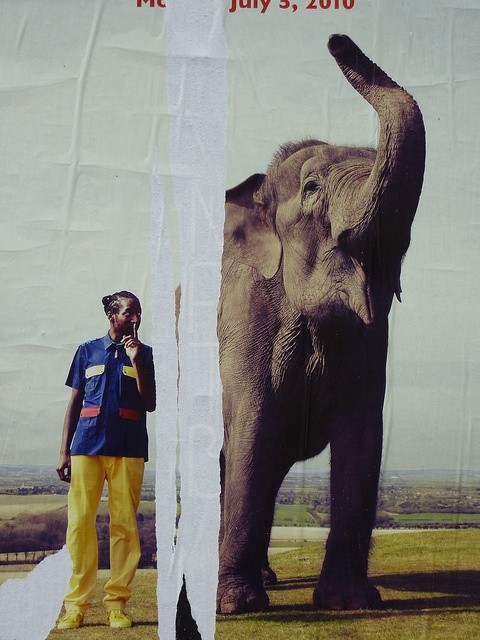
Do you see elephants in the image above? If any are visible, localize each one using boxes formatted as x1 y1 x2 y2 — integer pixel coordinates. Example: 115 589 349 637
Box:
178 31 427 618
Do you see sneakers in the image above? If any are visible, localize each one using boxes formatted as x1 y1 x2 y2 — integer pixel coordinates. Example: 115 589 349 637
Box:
105 609 132 628
57 610 85 630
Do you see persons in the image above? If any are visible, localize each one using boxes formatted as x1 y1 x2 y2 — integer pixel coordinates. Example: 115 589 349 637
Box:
55 290 156 630
